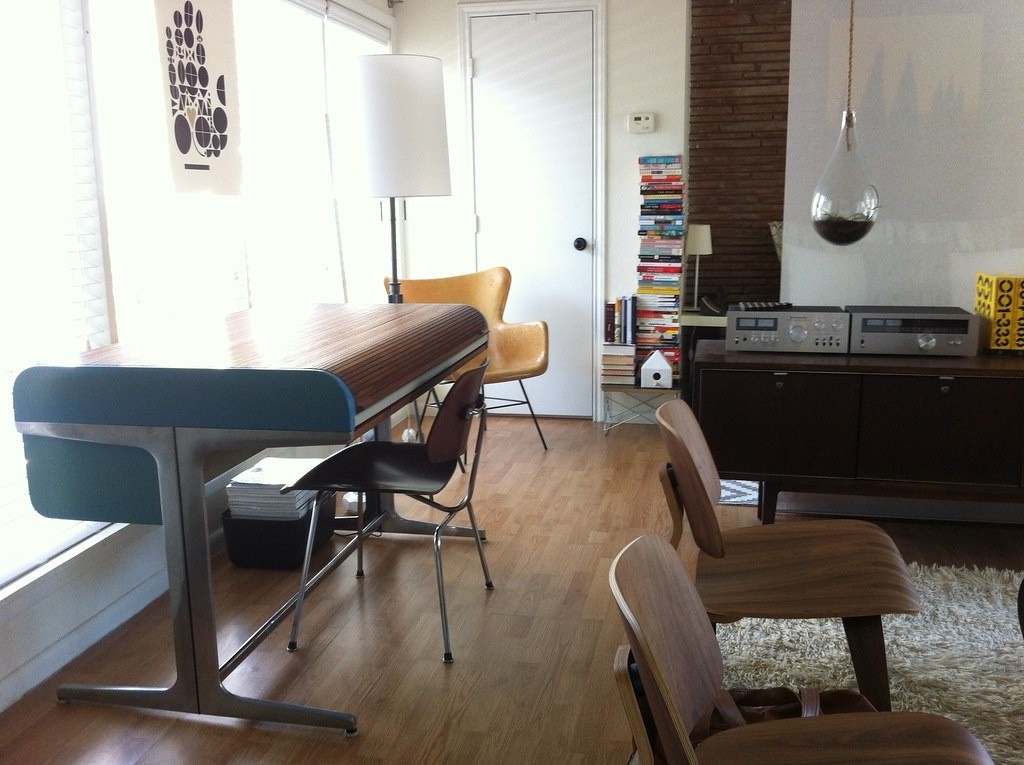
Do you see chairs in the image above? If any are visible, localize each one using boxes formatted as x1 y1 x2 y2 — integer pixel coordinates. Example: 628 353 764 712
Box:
607 531 994 764
384 266 549 473
655 399 920 710
279 356 495 663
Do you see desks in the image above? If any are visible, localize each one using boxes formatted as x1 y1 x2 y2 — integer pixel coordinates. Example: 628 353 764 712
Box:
601 386 683 435
13 302 491 738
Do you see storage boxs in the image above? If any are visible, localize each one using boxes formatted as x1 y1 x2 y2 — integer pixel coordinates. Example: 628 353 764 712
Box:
222 490 337 569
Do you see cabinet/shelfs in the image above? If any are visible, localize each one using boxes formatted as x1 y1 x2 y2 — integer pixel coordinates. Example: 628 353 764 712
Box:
691 338 1024 524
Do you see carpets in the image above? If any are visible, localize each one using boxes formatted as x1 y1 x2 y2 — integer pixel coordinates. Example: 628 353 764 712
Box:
716 562 1023 764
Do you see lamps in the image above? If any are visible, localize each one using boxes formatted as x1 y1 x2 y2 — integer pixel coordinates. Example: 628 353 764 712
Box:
682 223 713 310
351 54 467 304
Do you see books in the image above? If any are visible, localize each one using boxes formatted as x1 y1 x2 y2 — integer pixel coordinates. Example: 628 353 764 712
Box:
225 456 337 521
600 292 637 388
636 154 687 386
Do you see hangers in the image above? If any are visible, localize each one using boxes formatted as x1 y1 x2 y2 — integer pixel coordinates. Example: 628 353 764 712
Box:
717 479 758 507
